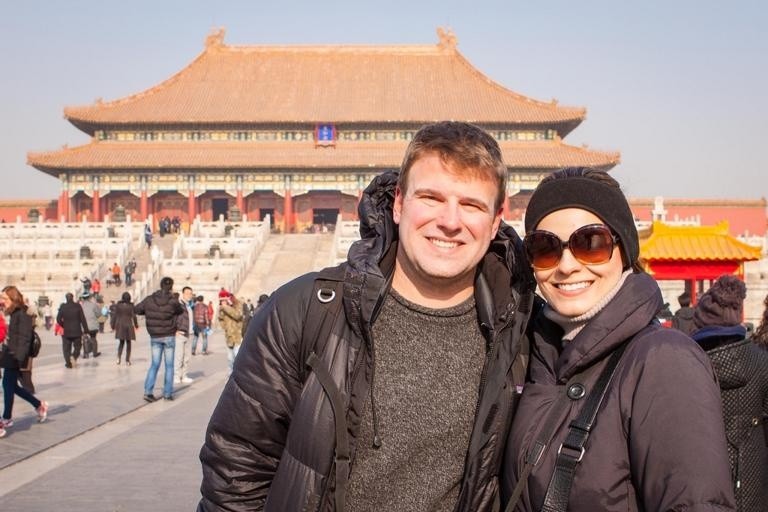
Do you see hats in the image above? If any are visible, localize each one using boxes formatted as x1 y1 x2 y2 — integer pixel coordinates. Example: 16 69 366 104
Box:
218 287 232 300
693 276 746 328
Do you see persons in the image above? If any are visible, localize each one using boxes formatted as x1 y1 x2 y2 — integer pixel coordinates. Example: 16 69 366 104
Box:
144 213 182 250
2 218 6 228
1 286 51 428
497 166 738 512
271 217 337 235
658 274 767 512
80 261 136 293
175 288 195 383
54 292 106 368
196 119 538 511
218 288 243 370
134 277 184 403
240 294 267 337
109 292 138 366
189 294 213 355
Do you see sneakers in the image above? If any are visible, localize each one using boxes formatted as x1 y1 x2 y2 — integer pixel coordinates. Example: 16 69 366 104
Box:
36 401 48 422
0 418 13 427
65 351 100 368
144 350 207 402
116 358 131 366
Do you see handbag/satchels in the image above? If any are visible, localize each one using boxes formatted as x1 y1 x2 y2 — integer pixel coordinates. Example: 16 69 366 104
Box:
83 334 92 353
30 330 40 357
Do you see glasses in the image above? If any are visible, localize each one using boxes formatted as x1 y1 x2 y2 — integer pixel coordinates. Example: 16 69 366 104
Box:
522 224 620 270
